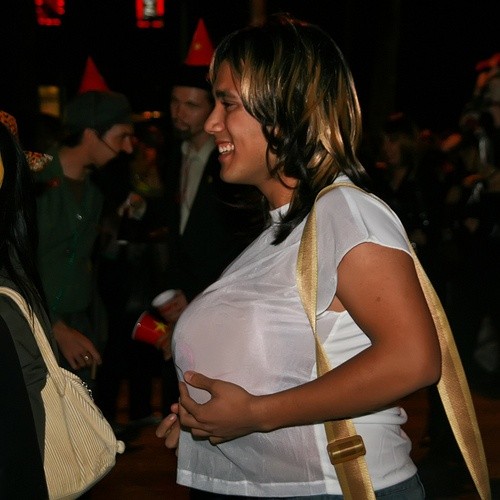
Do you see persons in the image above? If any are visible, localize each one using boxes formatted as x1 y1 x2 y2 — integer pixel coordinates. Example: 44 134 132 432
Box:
1 63 273 500
156 18 443 499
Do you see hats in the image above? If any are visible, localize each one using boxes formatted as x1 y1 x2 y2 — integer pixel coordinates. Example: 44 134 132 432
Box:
382 112 415 138
0 111 54 172
170 62 213 92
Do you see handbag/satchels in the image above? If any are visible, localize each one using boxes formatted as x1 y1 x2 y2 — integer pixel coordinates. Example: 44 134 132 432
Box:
0 285 126 500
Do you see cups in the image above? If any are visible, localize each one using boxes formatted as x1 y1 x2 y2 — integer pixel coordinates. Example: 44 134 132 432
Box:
130 312 171 346
151 289 187 321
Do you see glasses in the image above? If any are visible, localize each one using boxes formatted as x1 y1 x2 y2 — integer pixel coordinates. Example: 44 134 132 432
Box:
264 12 307 48
